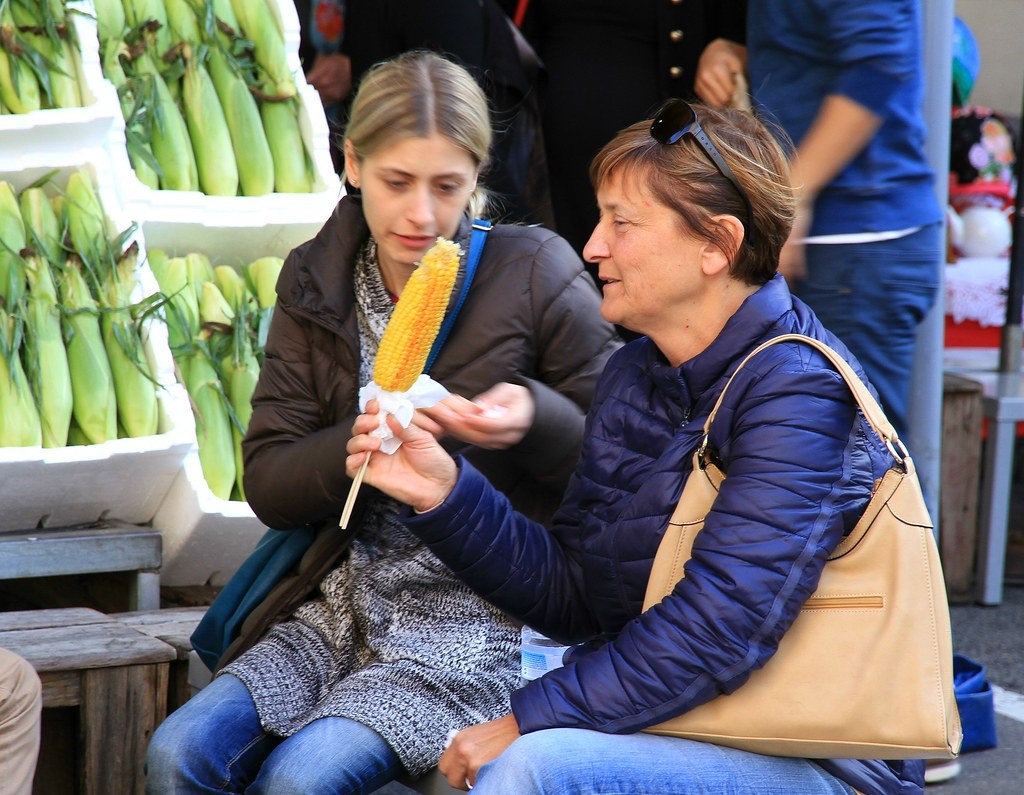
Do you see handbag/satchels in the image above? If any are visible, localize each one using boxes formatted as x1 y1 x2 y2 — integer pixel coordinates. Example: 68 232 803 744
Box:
638 334 963 759
190 526 317 673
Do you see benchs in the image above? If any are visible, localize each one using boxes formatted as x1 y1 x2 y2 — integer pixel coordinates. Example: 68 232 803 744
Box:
0 600 210 795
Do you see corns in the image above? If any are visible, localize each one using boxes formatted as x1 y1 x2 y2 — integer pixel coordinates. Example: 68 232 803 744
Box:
93 0 311 196
146 248 288 501
0 0 85 111
372 237 461 392
0 169 160 450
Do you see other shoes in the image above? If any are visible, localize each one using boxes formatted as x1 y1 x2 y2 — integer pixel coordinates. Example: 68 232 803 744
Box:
922 760 962 783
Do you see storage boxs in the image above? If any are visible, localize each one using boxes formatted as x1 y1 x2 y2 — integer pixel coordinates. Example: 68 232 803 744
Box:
1 0 354 585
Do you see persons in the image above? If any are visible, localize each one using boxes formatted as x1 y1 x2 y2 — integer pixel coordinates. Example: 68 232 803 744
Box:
293 0 944 516
0 647 43 795
141 53 627 795
345 98 927 795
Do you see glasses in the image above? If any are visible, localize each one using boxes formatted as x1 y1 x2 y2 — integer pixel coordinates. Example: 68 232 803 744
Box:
649 97 756 247
